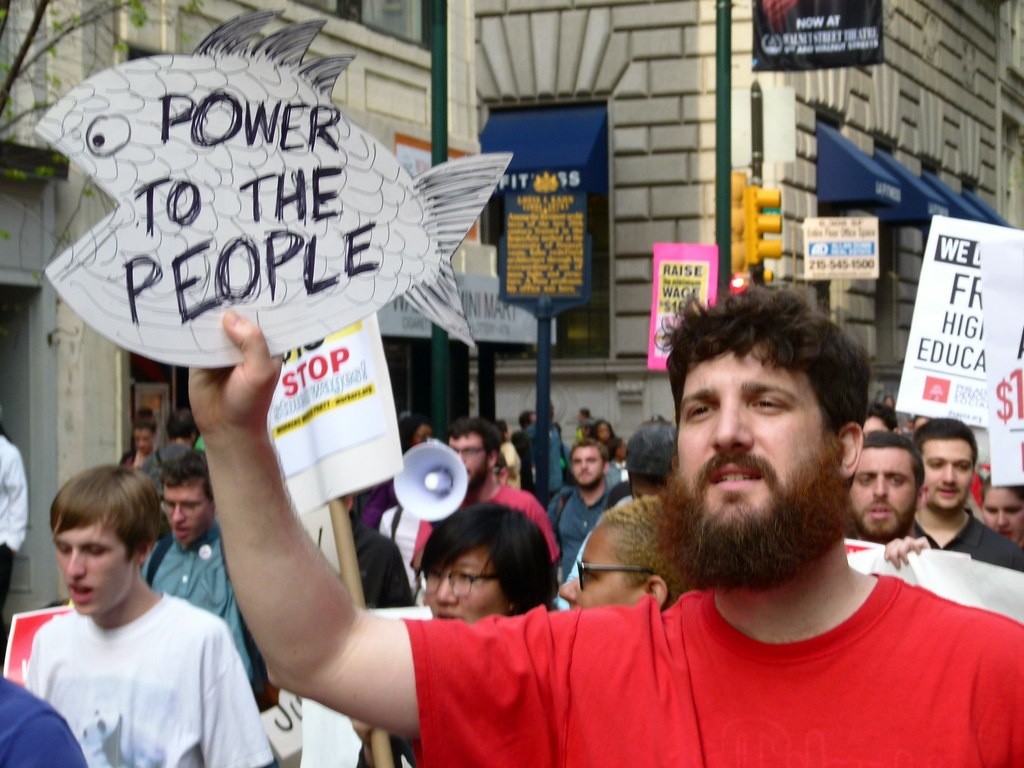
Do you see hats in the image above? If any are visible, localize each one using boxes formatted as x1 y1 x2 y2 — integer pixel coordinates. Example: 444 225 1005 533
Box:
625 423 676 478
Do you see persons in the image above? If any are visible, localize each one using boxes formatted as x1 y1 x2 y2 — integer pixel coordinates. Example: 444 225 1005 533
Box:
0 290 1024 768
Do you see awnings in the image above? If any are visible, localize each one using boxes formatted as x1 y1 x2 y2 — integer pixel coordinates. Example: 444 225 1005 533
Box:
816 120 1014 228
478 103 611 193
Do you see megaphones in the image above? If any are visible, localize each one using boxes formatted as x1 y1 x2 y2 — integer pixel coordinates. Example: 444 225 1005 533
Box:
394 443 468 522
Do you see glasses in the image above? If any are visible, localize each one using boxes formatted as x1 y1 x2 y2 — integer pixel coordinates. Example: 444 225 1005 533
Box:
160 498 208 516
576 561 672 599
455 447 484 457
415 567 499 598
